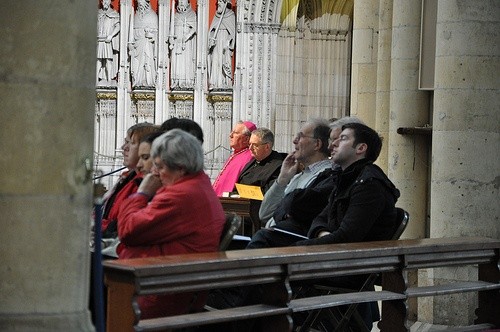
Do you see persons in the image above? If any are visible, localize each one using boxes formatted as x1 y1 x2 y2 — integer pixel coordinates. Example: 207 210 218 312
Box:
230 128 290 196
208 0 235 91
212 120 257 197
169 0 197 89
128 0 158 87
116 129 228 321
92 118 203 256
98 0 120 81
204 122 398 332
257 117 335 229
271 115 365 234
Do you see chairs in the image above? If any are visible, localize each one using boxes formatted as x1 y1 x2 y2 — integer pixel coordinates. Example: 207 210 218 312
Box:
296 207 409 332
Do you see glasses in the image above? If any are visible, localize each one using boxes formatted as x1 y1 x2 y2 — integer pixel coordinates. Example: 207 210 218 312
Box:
296 132 317 140
248 142 269 148
327 138 335 145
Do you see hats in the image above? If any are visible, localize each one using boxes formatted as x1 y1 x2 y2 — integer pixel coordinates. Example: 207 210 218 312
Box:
242 120 256 132
